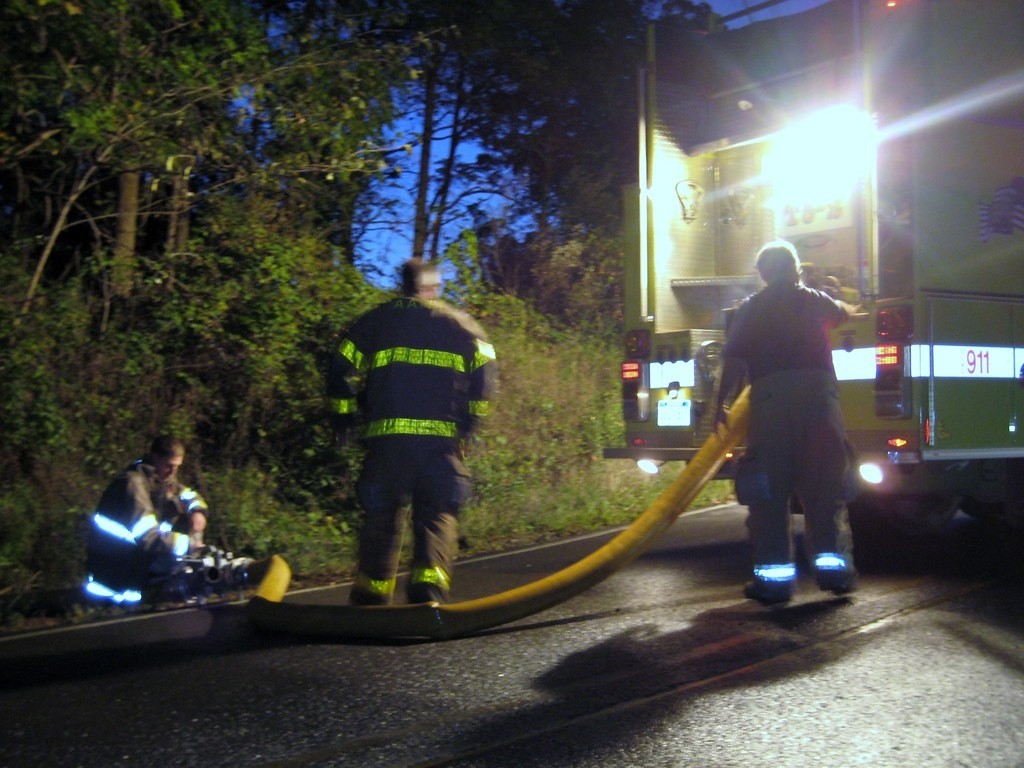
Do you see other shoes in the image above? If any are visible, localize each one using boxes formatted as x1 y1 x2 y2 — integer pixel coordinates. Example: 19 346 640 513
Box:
818 569 853 592
744 581 790 604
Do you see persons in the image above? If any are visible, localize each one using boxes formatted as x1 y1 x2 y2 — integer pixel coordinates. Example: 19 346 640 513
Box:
714 241 852 605
322 256 498 604
81 433 207 601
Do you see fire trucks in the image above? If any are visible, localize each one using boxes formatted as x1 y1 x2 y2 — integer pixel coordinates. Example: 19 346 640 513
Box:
603 0 1024 587
